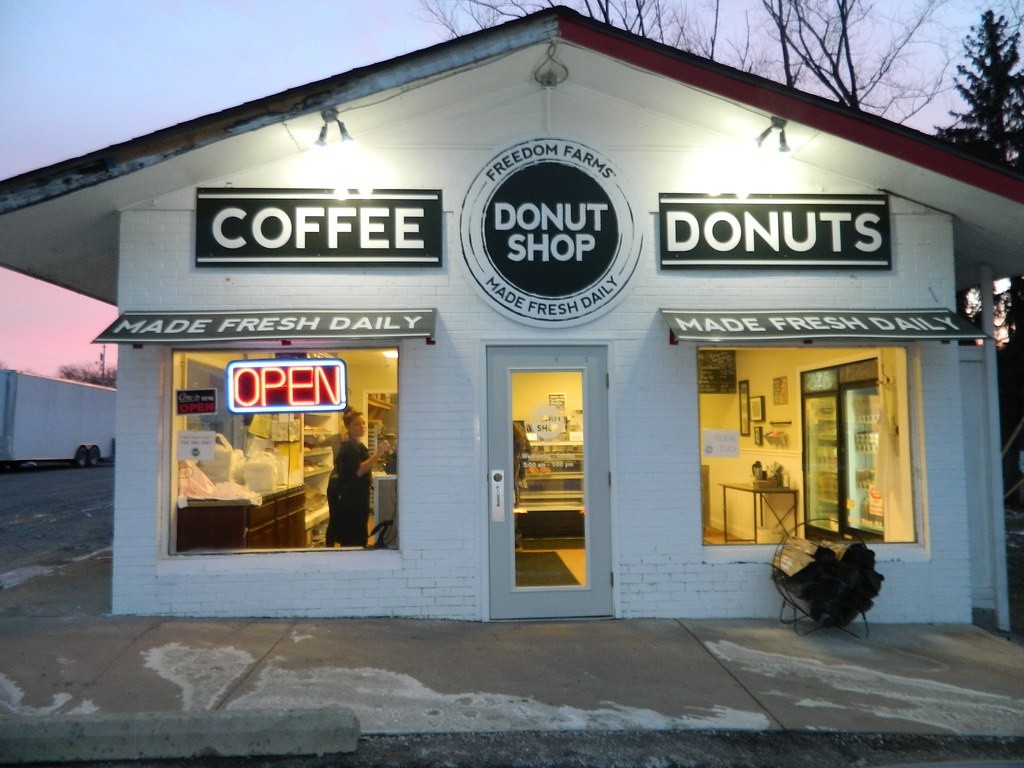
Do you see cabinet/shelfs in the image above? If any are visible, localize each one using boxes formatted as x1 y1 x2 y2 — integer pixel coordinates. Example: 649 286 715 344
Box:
303 412 339 532
518 441 585 511
177 485 305 552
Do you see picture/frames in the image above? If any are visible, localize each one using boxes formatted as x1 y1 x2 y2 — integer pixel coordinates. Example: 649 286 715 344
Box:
754 426 762 445
739 380 750 437
749 396 765 421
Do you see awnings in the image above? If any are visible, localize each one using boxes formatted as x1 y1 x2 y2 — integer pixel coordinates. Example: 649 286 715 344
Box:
661 306 990 342
92 307 436 347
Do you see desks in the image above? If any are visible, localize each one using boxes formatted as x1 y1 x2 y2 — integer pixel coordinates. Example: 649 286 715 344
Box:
718 483 799 544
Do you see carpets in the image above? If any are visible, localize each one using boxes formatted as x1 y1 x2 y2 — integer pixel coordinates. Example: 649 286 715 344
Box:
515 551 581 587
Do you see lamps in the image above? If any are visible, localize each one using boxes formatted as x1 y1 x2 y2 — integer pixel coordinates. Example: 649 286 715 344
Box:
315 108 355 149
753 116 790 153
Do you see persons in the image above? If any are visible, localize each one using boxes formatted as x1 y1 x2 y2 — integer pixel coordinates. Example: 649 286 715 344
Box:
385 433 398 474
514 423 529 506
305 422 343 547
336 407 389 547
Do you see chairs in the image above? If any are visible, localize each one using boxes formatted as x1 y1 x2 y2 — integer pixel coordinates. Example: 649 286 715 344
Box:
773 518 871 639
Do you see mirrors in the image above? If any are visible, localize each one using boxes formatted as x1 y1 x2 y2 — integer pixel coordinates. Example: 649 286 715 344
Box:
361 389 398 509
